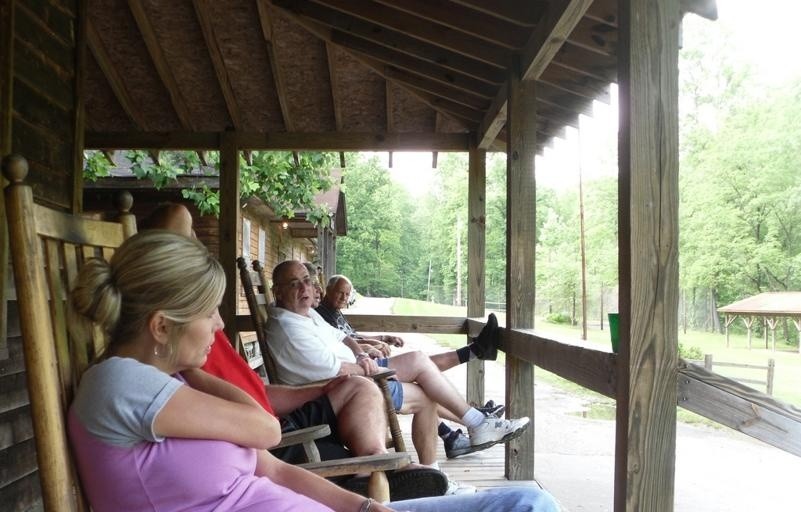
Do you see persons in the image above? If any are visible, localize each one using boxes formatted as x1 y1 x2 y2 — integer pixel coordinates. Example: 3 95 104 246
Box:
315 272 500 371
62 231 566 512
305 260 505 458
141 199 450 503
263 258 532 492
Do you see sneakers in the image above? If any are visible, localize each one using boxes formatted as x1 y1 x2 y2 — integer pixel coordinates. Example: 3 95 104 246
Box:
467 411 530 452
484 399 497 408
472 314 498 360
440 467 478 497
444 429 472 459
469 402 506 417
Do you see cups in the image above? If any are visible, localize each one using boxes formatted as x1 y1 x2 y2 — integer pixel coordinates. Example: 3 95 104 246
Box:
607 313 619 356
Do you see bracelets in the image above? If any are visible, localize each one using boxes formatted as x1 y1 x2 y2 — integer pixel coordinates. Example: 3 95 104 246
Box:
359 496 376 512
380 334 383 340
357 352 370 358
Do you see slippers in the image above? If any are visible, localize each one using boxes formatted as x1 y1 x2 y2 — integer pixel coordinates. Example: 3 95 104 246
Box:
343 462 449 503
